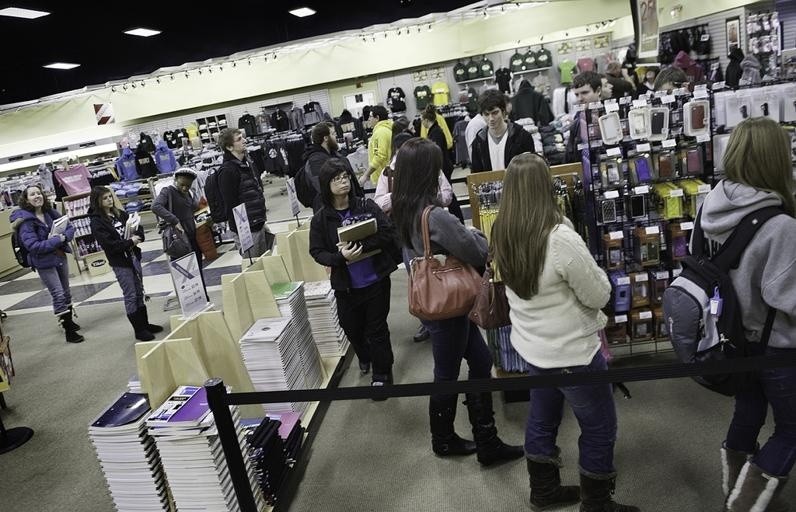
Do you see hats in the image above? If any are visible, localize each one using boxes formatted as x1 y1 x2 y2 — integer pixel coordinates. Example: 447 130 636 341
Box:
174 168 197 180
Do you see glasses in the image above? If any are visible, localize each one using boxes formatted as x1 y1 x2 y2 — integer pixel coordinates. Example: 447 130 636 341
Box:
334 175 349 182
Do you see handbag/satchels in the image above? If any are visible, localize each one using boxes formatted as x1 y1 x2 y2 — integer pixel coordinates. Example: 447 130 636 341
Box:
408 254 482 321
163 223 192 258
467 267 510 329
204 167 224 223
294 167 317 207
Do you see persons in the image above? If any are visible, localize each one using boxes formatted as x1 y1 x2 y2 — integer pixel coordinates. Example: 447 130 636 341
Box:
564 46 794 163
150 168 210 304
392 137 530 466
89 186 165 340
10 185 87 341
686 117 795 509
491 152 646 510
116 148 138 178
134 145 158 177
203 129 274 260
289 47 555 387
154 141 176 172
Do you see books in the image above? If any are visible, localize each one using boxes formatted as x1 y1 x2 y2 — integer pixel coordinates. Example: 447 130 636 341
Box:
49 214 67 235
238 281 351 419
264 229 276 249
86 375 310 512
122 212 143 241
52 218 67 239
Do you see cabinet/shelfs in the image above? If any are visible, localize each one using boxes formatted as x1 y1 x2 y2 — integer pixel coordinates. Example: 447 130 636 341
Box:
61 188 112 275
194 114 229 149
112 177 160 226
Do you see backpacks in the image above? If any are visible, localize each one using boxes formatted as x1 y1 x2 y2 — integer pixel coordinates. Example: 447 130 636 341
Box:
12 209 56 268
662 205 790 396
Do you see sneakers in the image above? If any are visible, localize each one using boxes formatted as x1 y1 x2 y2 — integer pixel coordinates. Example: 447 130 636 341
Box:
358 359 369 373
414 324 429 341
371 382 384 393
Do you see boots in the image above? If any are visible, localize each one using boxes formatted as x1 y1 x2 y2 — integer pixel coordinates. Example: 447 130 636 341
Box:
430 403 477 456
578 462 639 511
525 446 581 512
127 304 162 340
721 441 788 512
466 391 524 462
56 304 83 342
